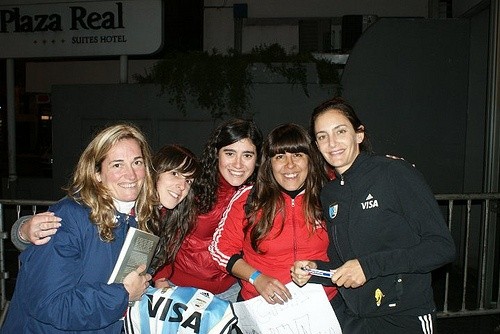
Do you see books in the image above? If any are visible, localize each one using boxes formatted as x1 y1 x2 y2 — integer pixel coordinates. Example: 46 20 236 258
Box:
107 226 159 307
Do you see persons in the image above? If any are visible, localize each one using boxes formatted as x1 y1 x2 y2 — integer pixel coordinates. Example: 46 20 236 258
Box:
7 123 152 333
10 117 337 334
290 99 455 334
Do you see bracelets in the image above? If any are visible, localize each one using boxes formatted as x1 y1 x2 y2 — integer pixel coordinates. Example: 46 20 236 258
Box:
249 270 262 285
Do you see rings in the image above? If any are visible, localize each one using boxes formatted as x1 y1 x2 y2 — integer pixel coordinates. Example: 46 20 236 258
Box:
271 294 276 299
